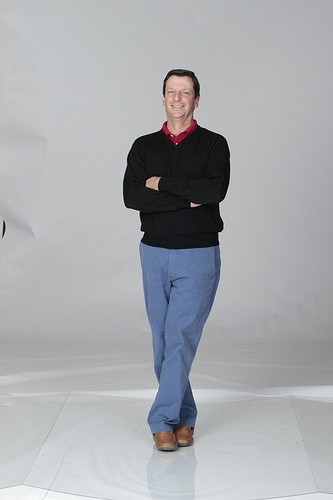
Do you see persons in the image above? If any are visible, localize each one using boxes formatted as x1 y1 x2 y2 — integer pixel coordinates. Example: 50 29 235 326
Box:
123 70 231 452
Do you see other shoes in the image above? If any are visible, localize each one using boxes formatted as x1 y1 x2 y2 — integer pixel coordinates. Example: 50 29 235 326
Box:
152 431 177 451
174 426 193 446
